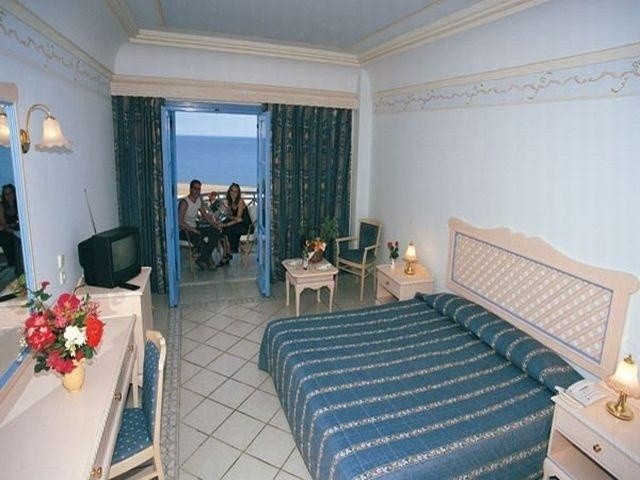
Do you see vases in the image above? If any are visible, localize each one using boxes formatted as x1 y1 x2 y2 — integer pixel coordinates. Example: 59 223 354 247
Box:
391 258 396 269
60 359 86 397
308 250 323 263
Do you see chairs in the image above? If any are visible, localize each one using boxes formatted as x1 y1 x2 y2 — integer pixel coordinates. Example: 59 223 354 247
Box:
334 218 384 302
107 329 169 480
239 199 258 266
176 198 195 273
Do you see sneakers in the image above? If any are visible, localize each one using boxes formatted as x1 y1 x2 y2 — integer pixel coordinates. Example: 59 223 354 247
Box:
207 258 216 270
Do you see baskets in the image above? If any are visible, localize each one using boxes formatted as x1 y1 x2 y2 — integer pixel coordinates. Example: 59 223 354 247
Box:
303 226 327 263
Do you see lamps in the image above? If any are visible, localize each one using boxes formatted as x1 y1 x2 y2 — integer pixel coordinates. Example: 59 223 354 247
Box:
403 240 417 275
21 103 75 153
1 114 11 148
604 353 639 421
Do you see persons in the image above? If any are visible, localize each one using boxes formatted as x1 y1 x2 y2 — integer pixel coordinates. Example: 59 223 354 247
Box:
215 183 254 267
1 183 24 276
178 179 221 271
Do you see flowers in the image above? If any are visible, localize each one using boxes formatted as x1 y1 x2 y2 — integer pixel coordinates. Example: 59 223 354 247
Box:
9 271 104 377
386 240 400 260
302 213 338 256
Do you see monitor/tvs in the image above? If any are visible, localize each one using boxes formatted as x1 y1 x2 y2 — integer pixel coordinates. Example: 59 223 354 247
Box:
12 230 26 289
78 226 141 288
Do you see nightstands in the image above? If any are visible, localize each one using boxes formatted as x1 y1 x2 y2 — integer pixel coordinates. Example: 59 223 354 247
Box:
375 263 435 306
542 380 639 479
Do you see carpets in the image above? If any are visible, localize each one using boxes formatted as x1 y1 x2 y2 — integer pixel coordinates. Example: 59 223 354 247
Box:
161 290 339 479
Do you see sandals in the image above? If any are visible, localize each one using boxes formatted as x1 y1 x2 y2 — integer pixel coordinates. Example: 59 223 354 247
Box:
218 253 232 266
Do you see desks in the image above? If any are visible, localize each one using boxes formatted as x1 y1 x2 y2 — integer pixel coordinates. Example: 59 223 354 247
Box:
74 266 153 387
1 312 137 480
281 256 339 317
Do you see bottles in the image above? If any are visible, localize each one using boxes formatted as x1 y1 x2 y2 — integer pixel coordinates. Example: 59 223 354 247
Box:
302 247 309 270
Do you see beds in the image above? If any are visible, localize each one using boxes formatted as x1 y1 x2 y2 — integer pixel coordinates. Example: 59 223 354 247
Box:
258 216 639 480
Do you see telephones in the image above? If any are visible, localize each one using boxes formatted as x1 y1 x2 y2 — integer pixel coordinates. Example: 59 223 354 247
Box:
566 378 609 407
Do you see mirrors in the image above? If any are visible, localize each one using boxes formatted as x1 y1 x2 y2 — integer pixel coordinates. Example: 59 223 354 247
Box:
1 78 41 427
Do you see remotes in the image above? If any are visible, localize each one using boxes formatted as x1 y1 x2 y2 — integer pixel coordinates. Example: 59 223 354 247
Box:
118 282 140 290
0 290 21 302
